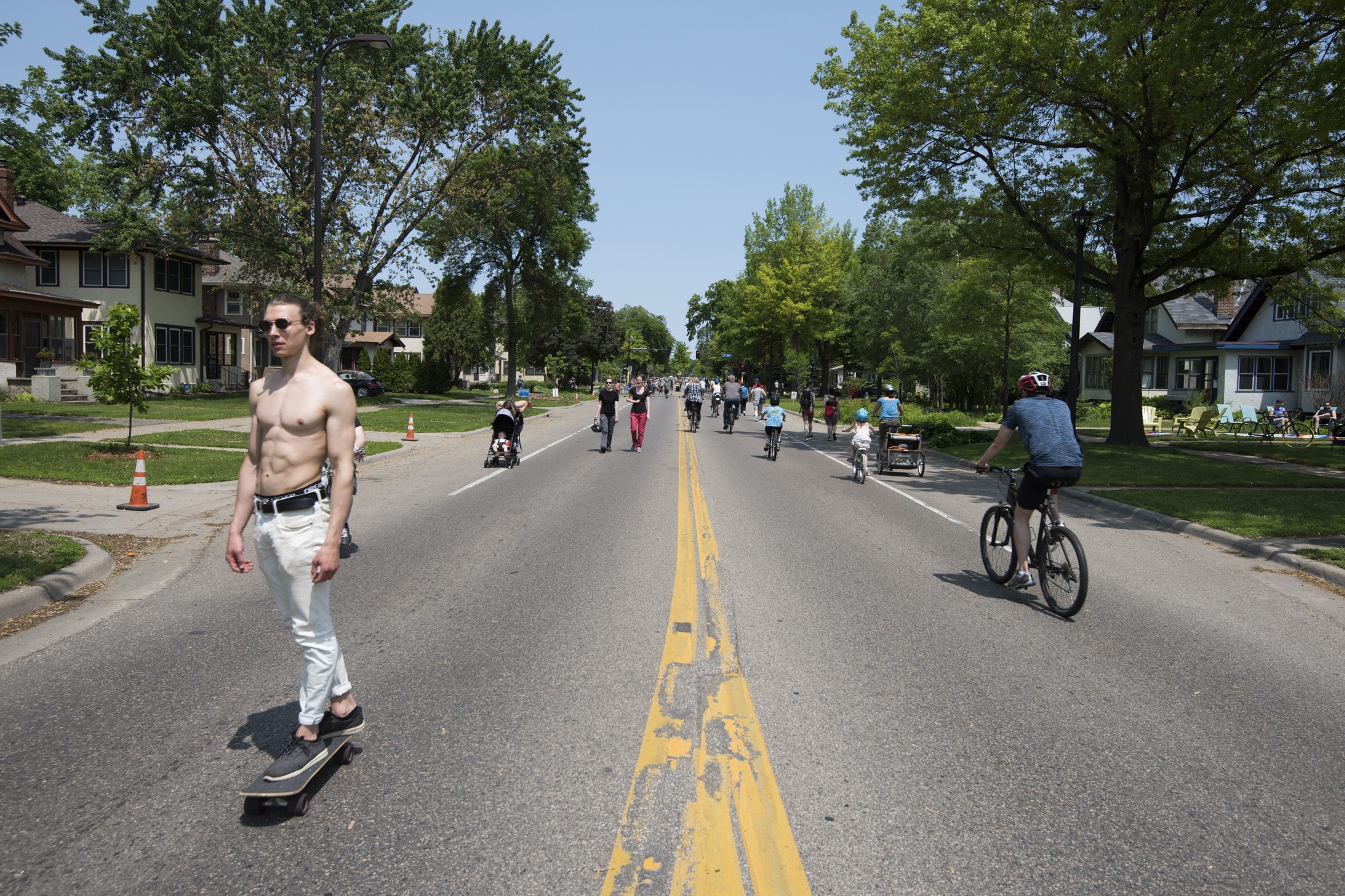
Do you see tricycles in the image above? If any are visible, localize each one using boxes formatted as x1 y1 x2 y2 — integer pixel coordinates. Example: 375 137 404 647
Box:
871 413 925 477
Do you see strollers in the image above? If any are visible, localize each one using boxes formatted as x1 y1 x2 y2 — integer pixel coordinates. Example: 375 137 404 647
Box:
484 406 523 469
675 383 681 392
648 384 653 395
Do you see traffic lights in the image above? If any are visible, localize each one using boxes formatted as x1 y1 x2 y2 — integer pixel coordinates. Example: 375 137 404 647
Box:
696 351 700 359
741 364 744 370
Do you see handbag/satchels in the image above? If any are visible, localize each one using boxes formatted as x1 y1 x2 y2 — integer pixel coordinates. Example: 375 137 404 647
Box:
825 406 835 417
759 389 766 404
627 384 629 387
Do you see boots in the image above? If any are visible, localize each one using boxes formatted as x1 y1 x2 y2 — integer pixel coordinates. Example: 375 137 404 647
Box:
495 450 509 459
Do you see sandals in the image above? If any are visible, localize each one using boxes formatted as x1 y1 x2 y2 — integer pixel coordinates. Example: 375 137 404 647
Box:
710 405 720 416
826 432 837 441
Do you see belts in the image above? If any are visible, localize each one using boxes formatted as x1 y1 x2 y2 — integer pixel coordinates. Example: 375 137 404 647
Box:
252 486 324 514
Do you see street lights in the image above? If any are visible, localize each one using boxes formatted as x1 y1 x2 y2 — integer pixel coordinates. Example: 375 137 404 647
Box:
625 329 640 388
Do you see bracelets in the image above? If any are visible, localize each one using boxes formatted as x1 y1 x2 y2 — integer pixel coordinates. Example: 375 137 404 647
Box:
839 415 841 416
751 398 753 399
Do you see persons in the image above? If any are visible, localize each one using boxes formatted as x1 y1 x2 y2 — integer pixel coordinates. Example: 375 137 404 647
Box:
226 294 356 781
823 387 841 441
835 388 840 400
975 372 1083 588
613 374 682 398
774 380 784 394
761 395 786 451
492 431 510 459
594 378 619 453
799 381 815 440
708 379 721 416
496 400 528 460
682 377 702 427
845 408 875 473
1269 399 1336 434
516 375 524 389
626 376 650 452
571 377 575 388
682 376 720 415
856 389 869 399
740 378 766 421
872 384 903 462
321 414 364 546
721 375 743 429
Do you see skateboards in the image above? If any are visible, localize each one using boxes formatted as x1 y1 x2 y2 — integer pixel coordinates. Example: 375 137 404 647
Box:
238 733 354 816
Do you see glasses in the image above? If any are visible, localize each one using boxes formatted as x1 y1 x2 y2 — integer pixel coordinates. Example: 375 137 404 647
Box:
606 382 613 384
258 318 307 332
616 381 617 382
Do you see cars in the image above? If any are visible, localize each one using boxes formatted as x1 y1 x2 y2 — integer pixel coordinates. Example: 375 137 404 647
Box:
337 369 385 397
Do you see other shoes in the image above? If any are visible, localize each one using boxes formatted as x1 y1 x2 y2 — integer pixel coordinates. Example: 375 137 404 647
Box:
665 396 668 398
687 413 700 429
755 419 761 421
741 412 746 416
847 455 870 476
764 439 783 451
631 444 642 453
671 393 673 395
723 415 739 429
600 447 611 453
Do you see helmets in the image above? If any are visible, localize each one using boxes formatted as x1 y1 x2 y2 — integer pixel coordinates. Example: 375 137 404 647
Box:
1018 372 1051 391
856 408 869 422
714 379 719 383
591 425 602 433
770 395 780 403
623 385 625 386
883 384 894 390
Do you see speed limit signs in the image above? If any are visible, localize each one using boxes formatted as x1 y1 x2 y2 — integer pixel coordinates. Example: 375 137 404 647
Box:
725 370 728 374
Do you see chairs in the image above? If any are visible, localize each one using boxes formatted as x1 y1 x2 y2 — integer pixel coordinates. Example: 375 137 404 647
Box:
1142 406 1162 432
1309 407 1338 436
1171 403 1288 439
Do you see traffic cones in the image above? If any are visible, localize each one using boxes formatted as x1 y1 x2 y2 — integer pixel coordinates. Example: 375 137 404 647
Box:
574 392 579 401
592 389 595 398
402 413 419 441
527 394 533 409
116 449 160 511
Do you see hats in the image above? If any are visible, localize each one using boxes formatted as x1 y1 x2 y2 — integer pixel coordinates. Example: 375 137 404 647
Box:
753 378 759 381
776 380 778 382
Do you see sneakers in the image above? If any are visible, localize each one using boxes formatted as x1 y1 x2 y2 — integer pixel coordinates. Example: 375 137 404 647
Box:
264 706 365 782
1007 520 1067 588
804 434 813 440
340 523 353 546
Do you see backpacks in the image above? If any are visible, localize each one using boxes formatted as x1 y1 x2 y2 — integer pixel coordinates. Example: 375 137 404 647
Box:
518 379 522 384
800 390 813 408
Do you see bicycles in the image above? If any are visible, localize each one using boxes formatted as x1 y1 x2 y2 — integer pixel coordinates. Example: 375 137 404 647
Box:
759 418 786 461
842 430 877 483
707 392 722 418
973 462 1089 617
1234 409 1314 448
720 398 743 435
914 389 929 403
681 396 704 433
